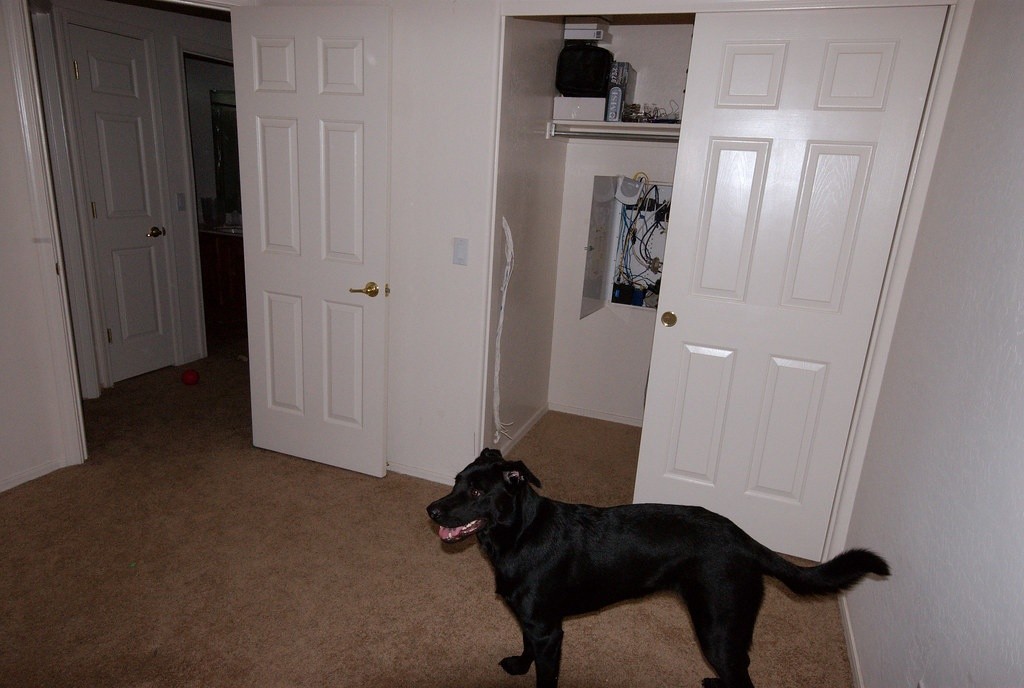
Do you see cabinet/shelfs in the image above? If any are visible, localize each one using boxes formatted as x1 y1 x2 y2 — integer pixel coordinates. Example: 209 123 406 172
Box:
628 4 953 564
199 233 246 323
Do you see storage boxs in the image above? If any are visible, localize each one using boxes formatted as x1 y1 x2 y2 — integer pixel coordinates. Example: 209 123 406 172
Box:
564 17 613 44
552 96 606 121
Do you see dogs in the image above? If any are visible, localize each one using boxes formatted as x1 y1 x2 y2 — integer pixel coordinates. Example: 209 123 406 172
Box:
426 448 893 688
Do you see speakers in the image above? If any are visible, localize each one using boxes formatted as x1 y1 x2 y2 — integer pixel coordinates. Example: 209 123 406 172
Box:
556 46 614 99
